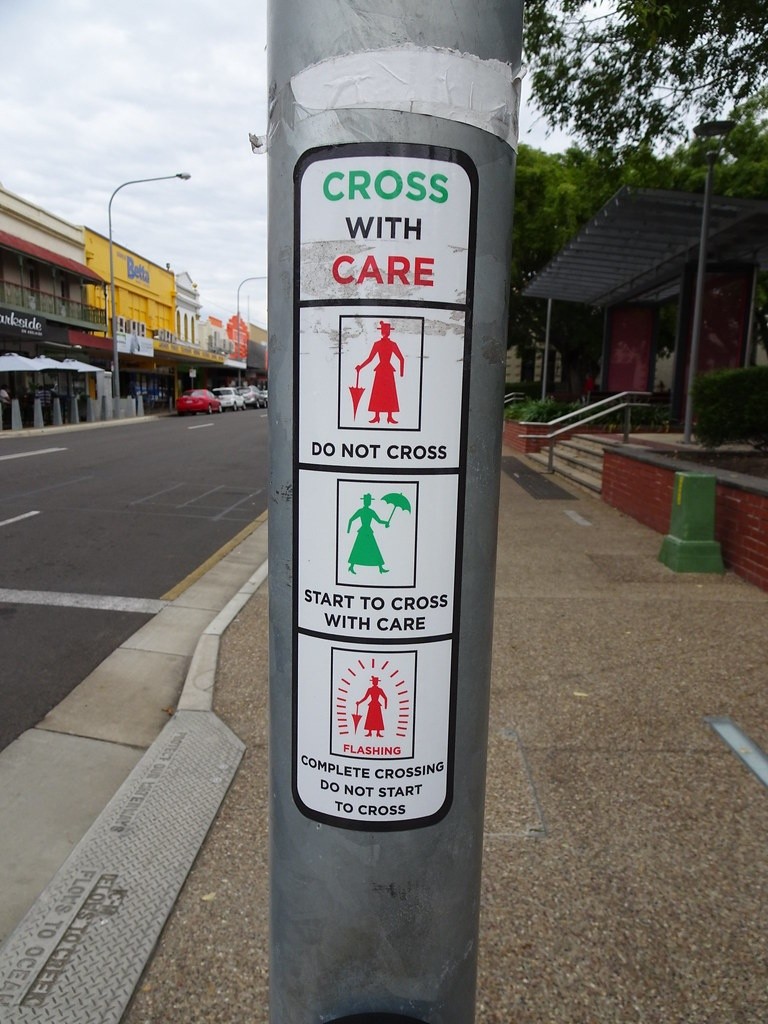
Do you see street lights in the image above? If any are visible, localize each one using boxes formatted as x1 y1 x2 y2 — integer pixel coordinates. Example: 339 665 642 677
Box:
109 171 193 419
682 120 738 445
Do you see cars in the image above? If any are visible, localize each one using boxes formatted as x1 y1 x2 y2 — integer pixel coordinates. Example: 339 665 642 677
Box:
176 389 223 416
236 385 267 410
211 386 247 412
258 388 268 408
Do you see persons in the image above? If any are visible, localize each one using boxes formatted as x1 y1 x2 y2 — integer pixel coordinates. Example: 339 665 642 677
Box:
45 384 50 425
34 385 44 425
0 384 12 426
